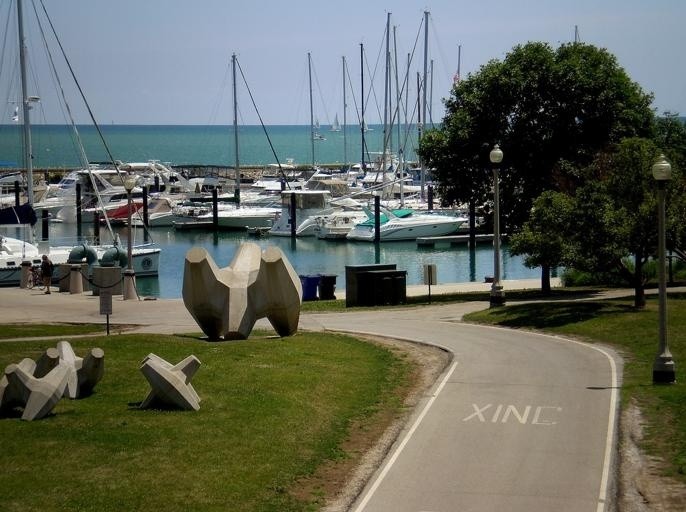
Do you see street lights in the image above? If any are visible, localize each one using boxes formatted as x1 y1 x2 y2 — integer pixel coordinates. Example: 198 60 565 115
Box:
653 154 676 386
489 144 506 308
122 175 135 270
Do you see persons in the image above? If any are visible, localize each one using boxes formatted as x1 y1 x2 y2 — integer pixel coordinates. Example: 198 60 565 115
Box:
37 255 54 294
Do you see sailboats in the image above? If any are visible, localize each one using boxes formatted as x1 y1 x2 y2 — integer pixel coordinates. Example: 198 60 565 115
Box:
0 0 162 287
0 6 476 237
345 11 468 242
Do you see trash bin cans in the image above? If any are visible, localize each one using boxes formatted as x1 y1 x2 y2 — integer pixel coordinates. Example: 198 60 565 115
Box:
299 273 320 301
319 274 338 300
